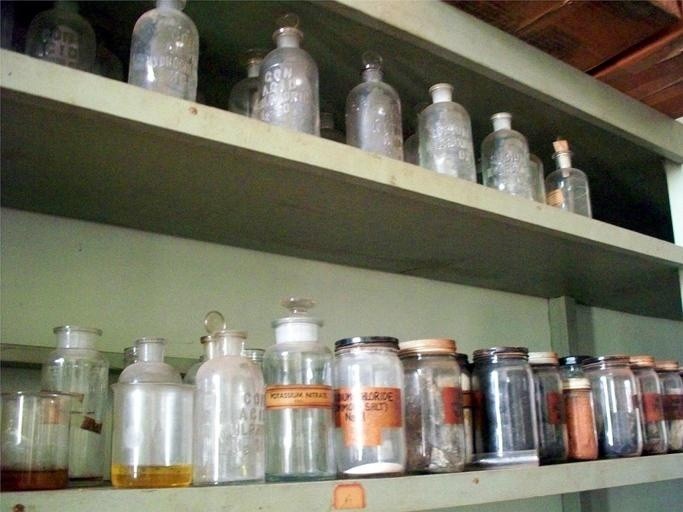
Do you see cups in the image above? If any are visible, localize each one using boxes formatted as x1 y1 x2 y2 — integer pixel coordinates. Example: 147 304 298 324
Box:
1 390 74 490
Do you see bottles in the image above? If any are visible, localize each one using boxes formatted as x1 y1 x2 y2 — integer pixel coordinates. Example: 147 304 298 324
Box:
0 2 594 220
42 296 682 490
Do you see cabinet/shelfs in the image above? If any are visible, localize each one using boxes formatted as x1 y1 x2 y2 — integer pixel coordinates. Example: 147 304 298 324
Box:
1 40 682 512
